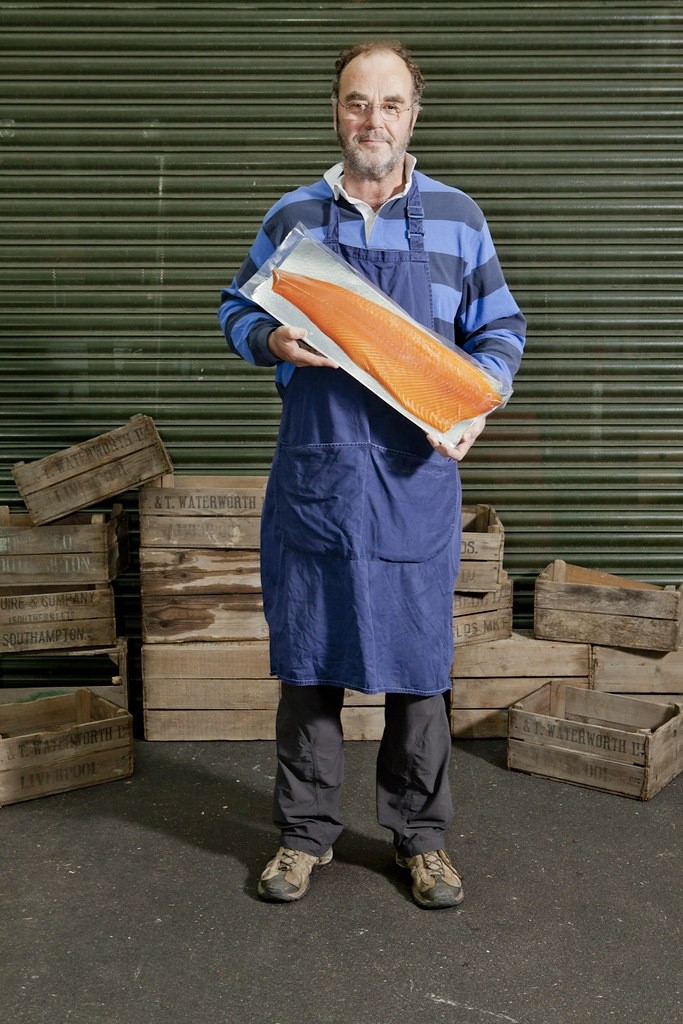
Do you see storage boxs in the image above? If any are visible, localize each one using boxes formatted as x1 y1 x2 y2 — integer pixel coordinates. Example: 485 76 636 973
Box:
0 412 682 810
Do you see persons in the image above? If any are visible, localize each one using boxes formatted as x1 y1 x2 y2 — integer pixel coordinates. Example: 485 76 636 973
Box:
216 40 533 910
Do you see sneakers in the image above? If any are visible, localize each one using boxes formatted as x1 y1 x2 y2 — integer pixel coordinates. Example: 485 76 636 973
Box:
259 845 334 902
396 849 463 908
